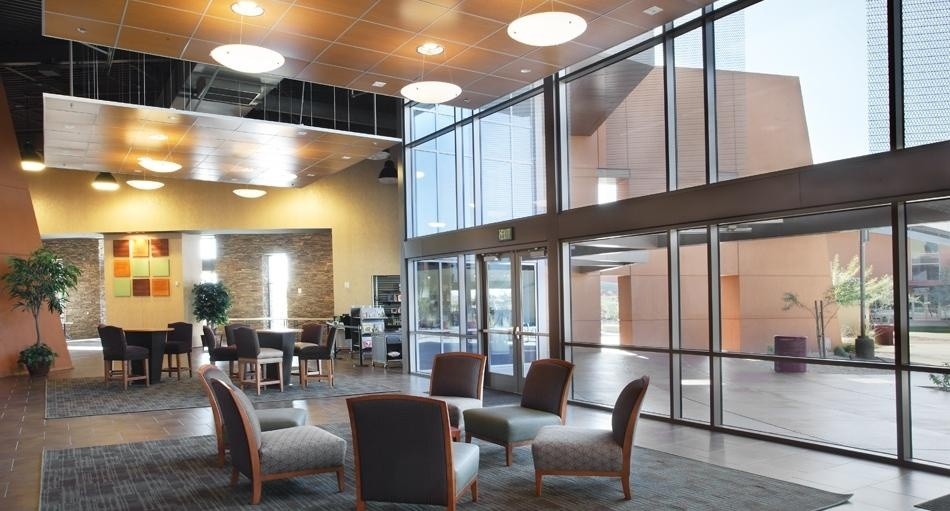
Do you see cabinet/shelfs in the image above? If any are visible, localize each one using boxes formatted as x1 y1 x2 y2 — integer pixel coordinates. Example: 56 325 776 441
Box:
376 287 403 326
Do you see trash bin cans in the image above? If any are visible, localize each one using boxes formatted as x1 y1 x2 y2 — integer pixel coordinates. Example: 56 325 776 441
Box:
875 325 893 345
775 336 806 372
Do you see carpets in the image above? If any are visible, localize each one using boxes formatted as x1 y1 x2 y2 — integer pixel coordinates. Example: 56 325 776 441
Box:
42 367 404 420
912 491 950 511
37 416 857 510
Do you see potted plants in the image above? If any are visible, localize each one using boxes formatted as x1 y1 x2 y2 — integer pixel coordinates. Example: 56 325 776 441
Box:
190 283 235 347
3 249 84 378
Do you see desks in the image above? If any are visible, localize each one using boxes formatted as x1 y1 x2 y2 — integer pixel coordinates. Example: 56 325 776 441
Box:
122 328 176 383
340 306 402 376
256 330 296 386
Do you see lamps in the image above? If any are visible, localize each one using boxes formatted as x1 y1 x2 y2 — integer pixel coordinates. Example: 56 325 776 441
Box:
136 132 183 173
398 42 464 108
367 146 392 163
377 157 400 185
126 154 164 190
19 89 47 172
505 0 587 50
92 170 119 191
205 0 286 77
232 181 267 197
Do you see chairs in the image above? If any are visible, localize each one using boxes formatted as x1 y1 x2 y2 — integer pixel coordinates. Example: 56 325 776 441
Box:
203 325 239 377
210 378 347 503
226 323 255 377
198 364 309 467
461 355 576 470
341 395 482 511
98 324 149 389
163 322 194 378
420 351 489 445
288 323 323 384
531 373 652 500
293 327 337 389
236 328 284 394
